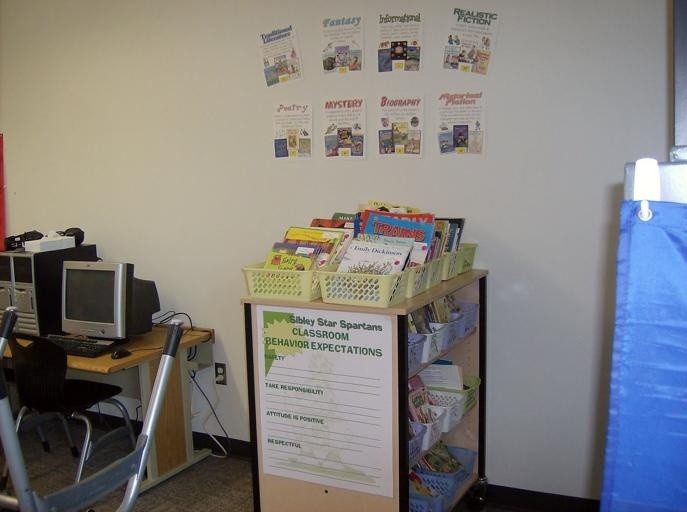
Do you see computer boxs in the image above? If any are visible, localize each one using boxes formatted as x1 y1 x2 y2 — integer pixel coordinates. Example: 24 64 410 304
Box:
0 244 97 337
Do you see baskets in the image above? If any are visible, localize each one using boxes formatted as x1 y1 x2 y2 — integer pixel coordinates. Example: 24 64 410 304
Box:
243 240 478 308
408 447 477 511
409 376 483 467
407 302 479 375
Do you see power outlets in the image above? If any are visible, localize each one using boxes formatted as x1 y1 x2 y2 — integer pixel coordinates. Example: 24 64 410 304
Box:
215 363 227 385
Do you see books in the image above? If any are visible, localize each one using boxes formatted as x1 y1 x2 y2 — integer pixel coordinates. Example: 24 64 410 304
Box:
405 295 471 501
263 200 465 303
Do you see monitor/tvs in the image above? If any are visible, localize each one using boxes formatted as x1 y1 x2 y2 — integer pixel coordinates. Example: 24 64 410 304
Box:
62 260 160 340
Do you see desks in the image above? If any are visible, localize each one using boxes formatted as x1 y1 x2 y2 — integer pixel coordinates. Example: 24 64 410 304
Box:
1 322 212 495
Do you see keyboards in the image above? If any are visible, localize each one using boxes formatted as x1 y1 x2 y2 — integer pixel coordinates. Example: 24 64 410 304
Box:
46 334 110 358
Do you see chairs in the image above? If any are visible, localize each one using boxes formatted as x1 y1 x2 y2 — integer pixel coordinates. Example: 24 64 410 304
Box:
0 333 137 493
0 306 184 512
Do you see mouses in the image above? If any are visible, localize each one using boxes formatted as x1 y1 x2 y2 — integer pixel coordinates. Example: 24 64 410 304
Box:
111 347 132 359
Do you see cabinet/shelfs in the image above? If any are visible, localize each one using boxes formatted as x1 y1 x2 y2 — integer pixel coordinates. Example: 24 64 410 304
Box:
240 270 490 508
